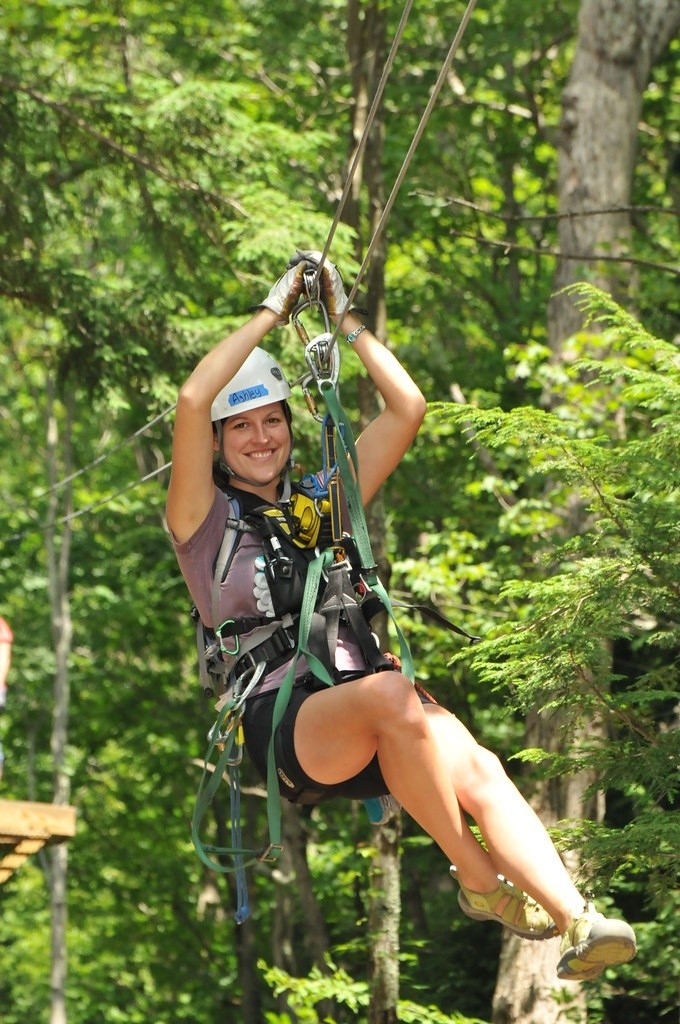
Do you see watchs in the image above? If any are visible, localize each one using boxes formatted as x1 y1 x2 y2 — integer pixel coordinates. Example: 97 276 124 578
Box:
346 325 366 344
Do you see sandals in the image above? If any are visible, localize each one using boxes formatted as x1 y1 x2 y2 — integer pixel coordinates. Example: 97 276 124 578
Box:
556 902 638 981
449 860 560 940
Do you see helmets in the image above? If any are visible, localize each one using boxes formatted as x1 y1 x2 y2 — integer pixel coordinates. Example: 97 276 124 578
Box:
209 346 293 422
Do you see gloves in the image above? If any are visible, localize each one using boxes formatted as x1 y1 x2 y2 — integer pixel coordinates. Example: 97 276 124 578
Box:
256 262 310 327
287 251 354 316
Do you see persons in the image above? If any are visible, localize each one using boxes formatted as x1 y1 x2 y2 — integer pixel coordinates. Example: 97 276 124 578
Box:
164 249 638 986
